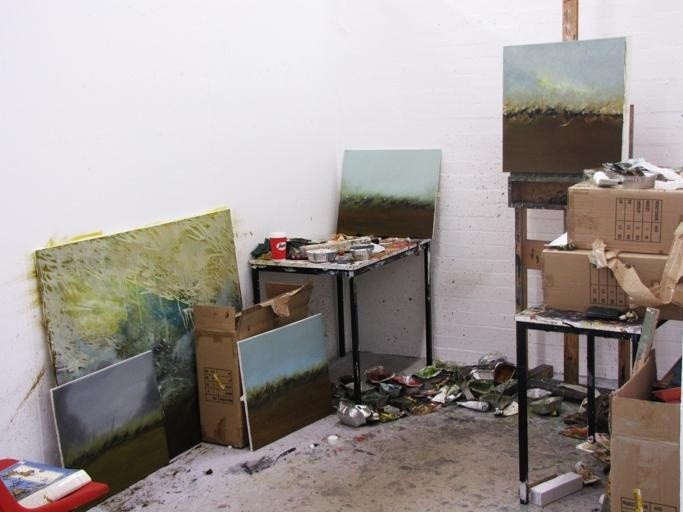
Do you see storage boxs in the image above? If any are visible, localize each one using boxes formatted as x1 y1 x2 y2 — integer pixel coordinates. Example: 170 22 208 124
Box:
610 358 680 511
540 234 683 319
567 176 683 254
194 280 312 448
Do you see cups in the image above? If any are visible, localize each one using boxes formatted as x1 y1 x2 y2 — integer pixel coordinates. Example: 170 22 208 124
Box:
268 231 289 263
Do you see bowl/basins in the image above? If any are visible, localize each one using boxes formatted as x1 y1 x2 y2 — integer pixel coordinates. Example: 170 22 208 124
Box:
349 245 376 261
304 249 338 264
621 170 656 189
336 363 562 426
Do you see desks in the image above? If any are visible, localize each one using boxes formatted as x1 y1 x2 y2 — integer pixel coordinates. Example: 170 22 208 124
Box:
514 307 641 506
249 240 433 406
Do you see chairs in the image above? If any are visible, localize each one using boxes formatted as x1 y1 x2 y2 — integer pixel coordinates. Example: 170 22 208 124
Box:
1 458 110 512
